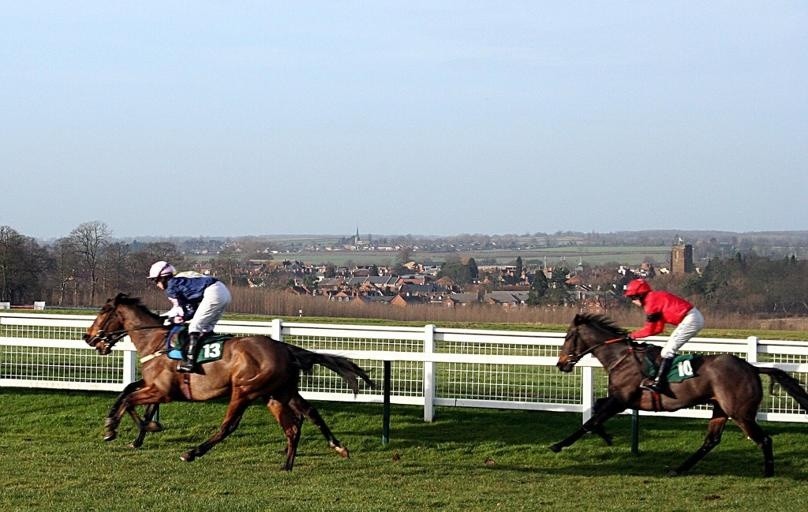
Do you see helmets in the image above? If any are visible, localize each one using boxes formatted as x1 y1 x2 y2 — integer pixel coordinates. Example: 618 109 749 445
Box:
623 279 650 297
147 260 174 280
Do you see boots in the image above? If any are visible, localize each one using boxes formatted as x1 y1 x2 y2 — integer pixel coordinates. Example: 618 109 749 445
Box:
176 332 201 372
643 358 671 392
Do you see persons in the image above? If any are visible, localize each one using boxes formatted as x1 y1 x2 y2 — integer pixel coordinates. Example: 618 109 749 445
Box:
147 260 232 372
624 278 705 391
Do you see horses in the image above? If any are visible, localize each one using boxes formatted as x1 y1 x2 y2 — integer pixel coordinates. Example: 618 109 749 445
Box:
82 291 376 474
547 311 808 479
